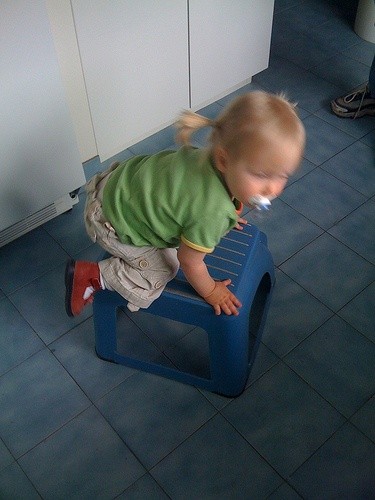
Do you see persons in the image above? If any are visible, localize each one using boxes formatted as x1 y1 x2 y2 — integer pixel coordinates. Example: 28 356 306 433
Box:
332 54 375 119
64 90 306 318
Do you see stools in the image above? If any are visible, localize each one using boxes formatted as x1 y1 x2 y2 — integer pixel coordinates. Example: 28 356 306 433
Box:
93 222 277 398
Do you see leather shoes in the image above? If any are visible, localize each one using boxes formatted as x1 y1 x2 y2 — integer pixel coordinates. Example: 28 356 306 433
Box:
64 260 101 320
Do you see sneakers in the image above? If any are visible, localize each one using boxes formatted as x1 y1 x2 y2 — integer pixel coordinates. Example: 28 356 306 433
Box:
330 84 375 121
